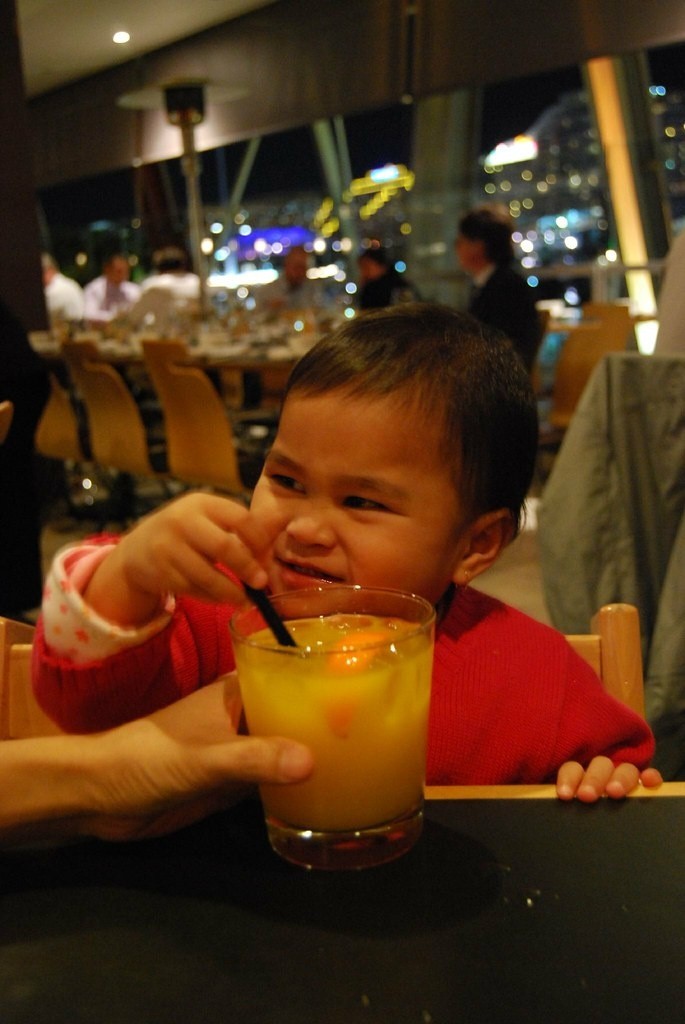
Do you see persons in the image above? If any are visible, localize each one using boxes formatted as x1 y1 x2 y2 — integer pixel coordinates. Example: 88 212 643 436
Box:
0 203 537 837
29 304 663 800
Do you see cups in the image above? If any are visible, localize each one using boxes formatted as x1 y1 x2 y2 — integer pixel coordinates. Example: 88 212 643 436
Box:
230 585 436 873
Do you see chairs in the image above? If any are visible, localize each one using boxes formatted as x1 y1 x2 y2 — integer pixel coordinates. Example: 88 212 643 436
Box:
548 302 630 428
59 339 179 522
138 339 257 504
37 373 94 522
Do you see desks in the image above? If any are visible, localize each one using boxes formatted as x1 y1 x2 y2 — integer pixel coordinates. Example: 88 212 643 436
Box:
0 781 685 1024
35 336 327 487
